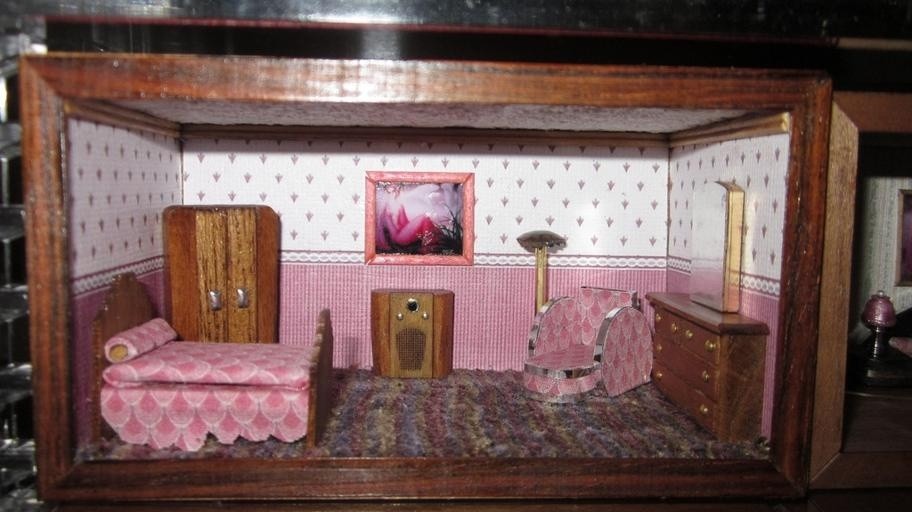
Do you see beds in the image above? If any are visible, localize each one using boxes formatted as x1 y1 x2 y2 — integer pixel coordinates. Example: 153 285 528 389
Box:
89 269 337 447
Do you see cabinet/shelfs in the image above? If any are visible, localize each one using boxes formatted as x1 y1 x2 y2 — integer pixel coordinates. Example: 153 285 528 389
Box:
644 290 769 443
162 203 282 343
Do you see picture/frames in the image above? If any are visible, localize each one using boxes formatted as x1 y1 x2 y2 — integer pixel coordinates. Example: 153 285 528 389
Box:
363 169 477 268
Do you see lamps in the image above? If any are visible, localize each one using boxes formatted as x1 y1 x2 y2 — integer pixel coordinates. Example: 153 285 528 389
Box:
858 289 899 369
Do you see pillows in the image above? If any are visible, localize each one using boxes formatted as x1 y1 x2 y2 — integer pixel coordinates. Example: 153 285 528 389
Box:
104 316 179 363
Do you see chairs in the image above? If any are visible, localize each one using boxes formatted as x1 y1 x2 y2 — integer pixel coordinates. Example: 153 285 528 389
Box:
521 285 656 406
